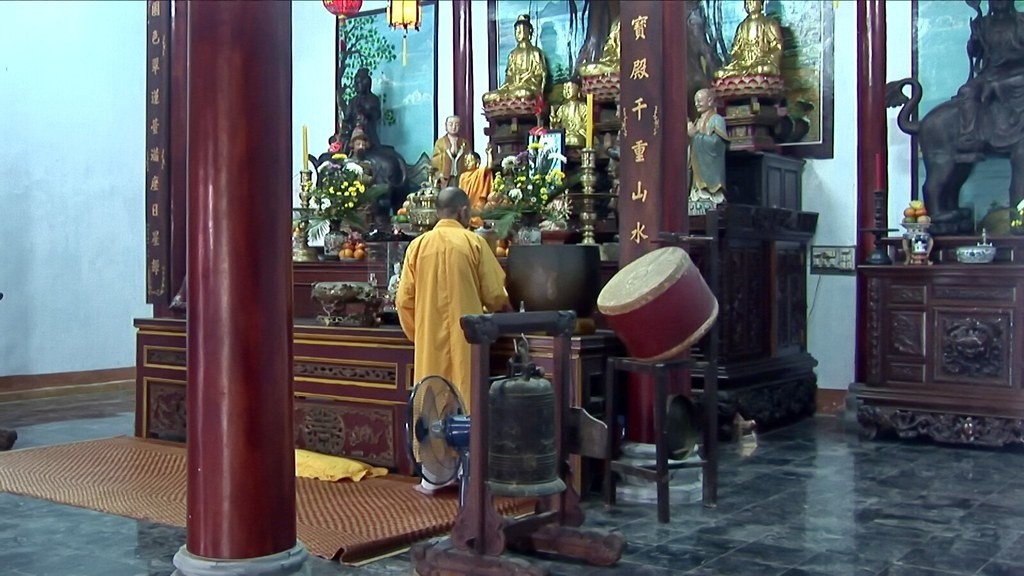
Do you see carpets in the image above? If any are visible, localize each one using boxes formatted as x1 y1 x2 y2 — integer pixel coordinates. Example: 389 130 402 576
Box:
0 434 522 568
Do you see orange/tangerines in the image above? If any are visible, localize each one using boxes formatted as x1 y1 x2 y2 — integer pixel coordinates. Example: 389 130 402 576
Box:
390 208 408 223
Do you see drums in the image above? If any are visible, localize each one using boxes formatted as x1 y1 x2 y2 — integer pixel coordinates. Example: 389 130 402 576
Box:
595 244 720 363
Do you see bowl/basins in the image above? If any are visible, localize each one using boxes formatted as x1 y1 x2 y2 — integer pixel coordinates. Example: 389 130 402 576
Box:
953 247 996 264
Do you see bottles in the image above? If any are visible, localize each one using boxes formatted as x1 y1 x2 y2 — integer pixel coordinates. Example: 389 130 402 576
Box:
902 222 931 254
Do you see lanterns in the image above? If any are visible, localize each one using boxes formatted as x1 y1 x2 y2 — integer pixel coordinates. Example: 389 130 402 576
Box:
386 0 422 67
323 0 363 52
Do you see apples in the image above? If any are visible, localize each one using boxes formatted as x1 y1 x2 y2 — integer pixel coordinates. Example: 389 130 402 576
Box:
484 193 512 209
339 240 367 259
904 200 932 222
495 239 509 257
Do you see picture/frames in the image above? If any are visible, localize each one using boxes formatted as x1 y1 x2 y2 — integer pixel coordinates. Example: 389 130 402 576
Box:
332 0 438 163
487 0 835 159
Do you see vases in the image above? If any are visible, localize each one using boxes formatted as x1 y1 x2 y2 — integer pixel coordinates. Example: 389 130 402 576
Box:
514 222 542 246
326 232 347 258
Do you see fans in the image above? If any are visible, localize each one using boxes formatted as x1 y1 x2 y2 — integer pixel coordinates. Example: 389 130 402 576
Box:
406 373 468 513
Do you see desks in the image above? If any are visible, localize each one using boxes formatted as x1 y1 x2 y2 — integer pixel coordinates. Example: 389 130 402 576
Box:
131 316 618 504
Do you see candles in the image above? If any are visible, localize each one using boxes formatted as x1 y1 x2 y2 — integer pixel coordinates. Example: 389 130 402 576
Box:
587 92 593 147
301 124 308 171
875 152 881 192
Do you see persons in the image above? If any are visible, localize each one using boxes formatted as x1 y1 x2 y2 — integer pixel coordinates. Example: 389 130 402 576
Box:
686 87 731 204
395 186 513 497
482 14 548 103
549 78 588 147
336 67 382 146
713 0 784 78
458 142 494 212
347 126 371 164
431 115 472 186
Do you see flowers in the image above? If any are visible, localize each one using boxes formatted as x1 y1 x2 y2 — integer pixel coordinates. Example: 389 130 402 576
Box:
308 155 366 222
492 139 567 222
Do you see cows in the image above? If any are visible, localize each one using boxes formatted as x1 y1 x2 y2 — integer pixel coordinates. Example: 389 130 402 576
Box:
309 144 410 218
